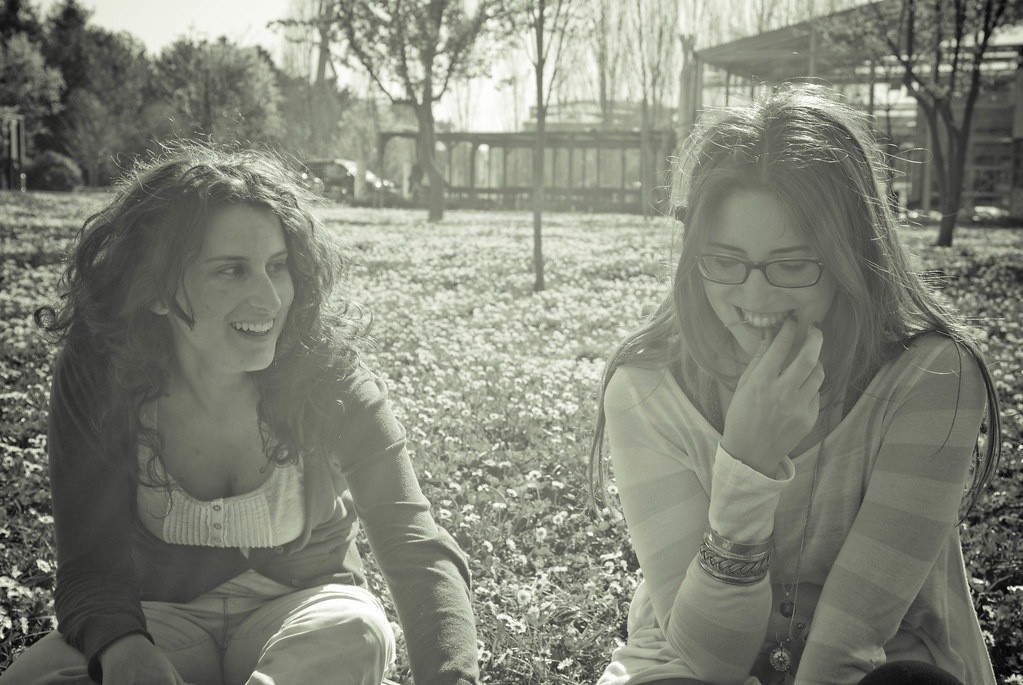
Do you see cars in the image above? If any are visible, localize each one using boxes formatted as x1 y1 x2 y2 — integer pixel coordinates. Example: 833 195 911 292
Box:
300 160 394 200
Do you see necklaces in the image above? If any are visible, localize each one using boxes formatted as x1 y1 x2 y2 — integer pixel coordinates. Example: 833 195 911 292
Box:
770 402 832 672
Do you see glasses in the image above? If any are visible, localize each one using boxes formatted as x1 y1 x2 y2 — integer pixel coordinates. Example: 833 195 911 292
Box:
693 253 826 289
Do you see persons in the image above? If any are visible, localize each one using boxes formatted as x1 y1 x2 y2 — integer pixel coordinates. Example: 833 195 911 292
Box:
596 99 1003 685
0 149 479 685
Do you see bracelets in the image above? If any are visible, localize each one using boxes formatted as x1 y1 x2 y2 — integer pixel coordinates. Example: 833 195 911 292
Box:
699 522 770 586
